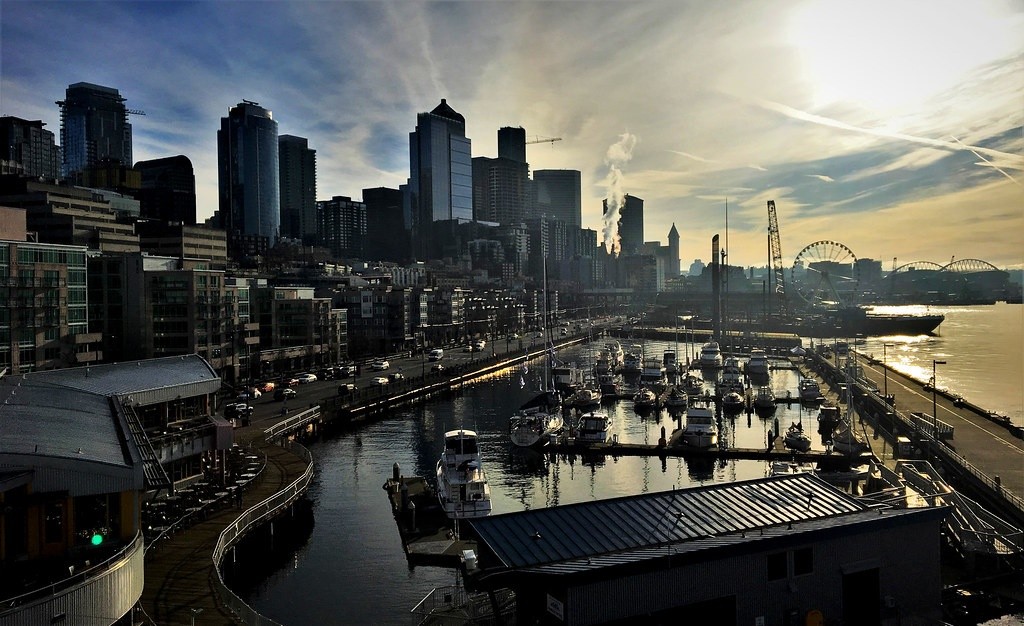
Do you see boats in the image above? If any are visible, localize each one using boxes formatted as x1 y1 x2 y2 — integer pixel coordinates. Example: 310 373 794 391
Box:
433 425 493 519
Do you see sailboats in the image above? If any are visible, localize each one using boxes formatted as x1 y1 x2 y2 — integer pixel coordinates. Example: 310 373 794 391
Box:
508 241 870 456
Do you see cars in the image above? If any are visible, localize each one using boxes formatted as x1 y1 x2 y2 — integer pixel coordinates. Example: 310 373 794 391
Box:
371 360 389 371
463 340 486 353
234 365 359 402
370 376 389 387
224 402 255 419
429 348 444 361
431 364 444 374
274 388 297 402
390 372 405 381
